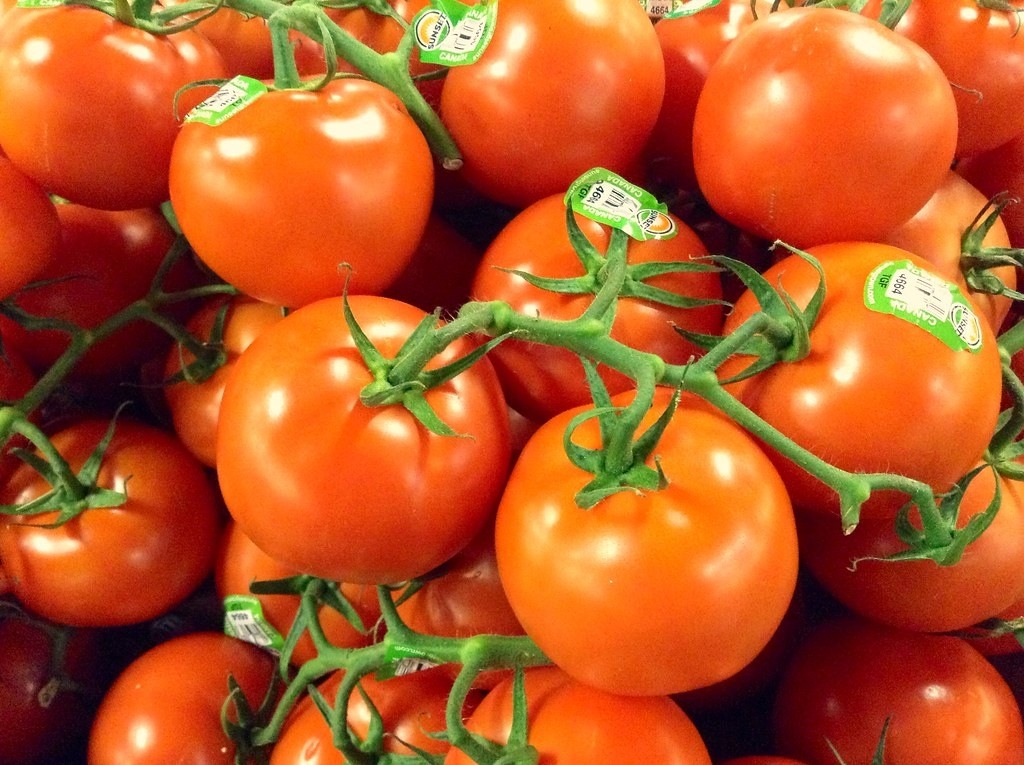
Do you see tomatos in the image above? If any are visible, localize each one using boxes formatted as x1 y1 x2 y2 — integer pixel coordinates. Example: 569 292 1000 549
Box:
0 0 1024 765
